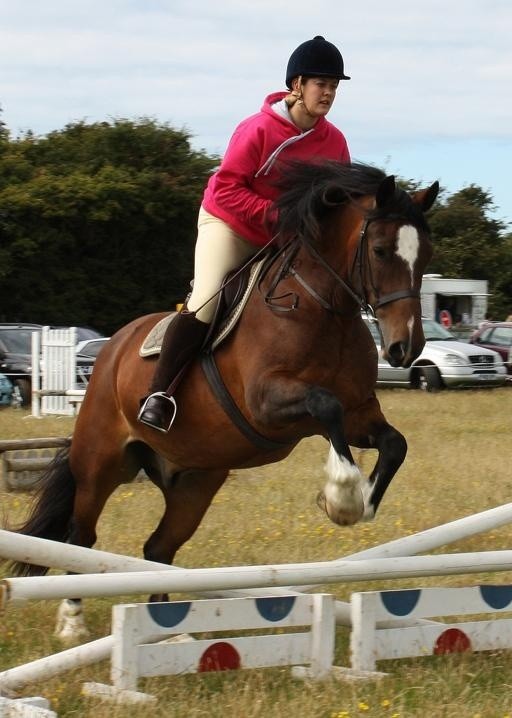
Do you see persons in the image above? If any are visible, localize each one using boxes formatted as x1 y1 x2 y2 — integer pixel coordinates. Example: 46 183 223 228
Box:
137 35 351 431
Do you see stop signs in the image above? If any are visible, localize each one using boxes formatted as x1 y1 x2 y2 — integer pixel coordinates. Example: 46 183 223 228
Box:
440 310 452 327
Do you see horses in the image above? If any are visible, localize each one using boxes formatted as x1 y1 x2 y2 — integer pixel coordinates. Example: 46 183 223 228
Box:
1 155 440 643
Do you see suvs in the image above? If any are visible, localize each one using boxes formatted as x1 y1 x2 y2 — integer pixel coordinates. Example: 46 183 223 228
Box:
0 322 95 409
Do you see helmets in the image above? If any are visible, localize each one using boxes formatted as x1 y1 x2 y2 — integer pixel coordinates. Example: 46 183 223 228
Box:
285 35 350 88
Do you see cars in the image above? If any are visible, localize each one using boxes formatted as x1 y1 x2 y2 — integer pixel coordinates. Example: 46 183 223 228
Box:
470 319 512 375
69 325 110 356
360 312 507 389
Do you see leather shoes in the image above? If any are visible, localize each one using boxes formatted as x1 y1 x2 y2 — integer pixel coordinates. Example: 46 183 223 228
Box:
141 396 165 427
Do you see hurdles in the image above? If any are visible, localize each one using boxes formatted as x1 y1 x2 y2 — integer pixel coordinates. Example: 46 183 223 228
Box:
0 437 149 492
0 504 512 717
